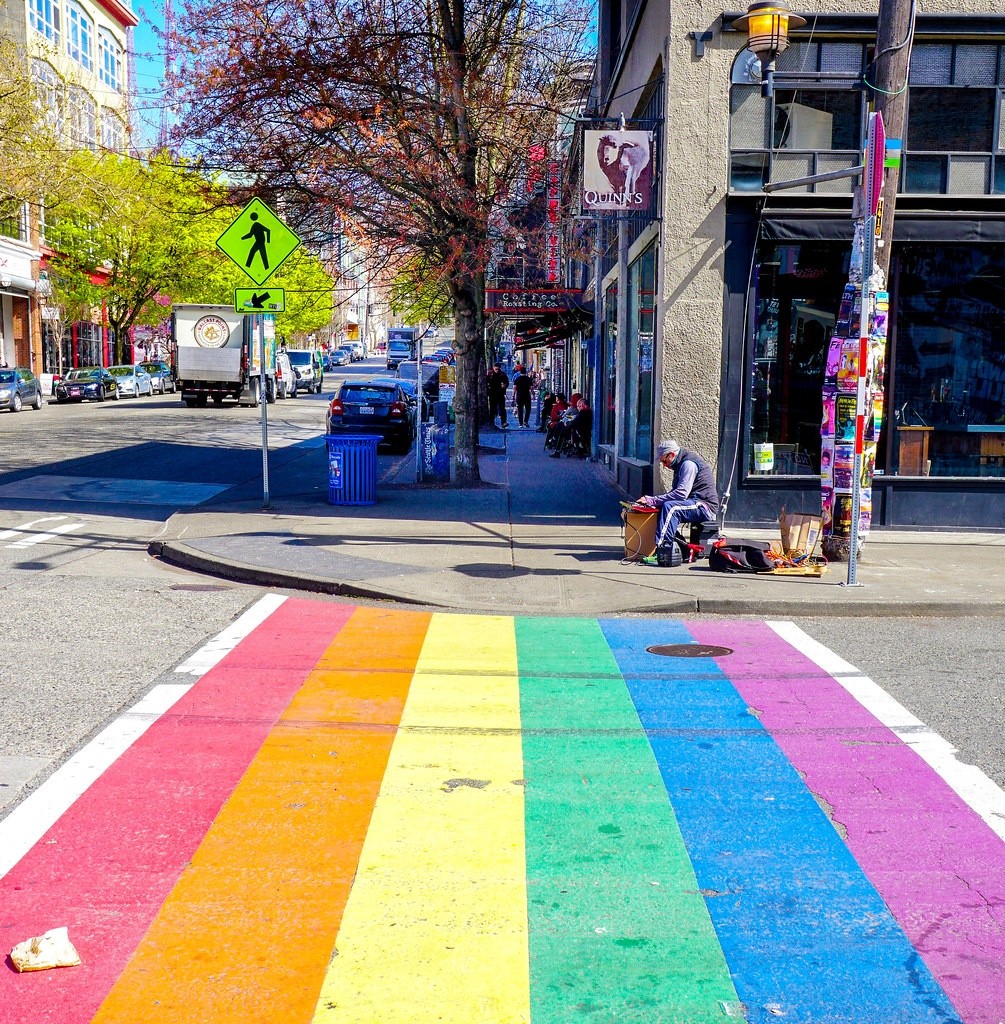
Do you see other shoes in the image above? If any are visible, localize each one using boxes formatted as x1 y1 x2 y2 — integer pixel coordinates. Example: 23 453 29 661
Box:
549 451 560 458
502 422 509 428
523 422 530 428
518 424 523 427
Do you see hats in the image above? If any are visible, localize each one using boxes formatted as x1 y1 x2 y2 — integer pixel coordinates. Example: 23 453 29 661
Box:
516 360 519 364
655 440 680 461
518 365 522 370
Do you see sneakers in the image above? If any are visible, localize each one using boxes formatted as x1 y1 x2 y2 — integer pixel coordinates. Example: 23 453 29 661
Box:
643 553 658 563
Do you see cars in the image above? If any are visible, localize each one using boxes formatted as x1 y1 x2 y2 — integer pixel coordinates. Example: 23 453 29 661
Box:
322 354 333 372
425 327 438 338
499 342 511 349
377 342 386 350
326 380 417 452
330 341 364 366
56 366 119 402
395 359 440 415
275 352 301 399
128 361 176 394
752 357 778 387
371 378 431 423
102 365 153 398
422 348 457 365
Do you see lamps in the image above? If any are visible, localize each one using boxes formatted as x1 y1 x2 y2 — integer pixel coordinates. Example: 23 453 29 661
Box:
731 0 808 98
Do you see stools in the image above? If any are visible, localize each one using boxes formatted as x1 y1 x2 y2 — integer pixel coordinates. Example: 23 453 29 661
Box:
690 520 720 557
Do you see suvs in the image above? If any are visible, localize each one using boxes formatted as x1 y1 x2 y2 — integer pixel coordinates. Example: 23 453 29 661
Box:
0 366 42 412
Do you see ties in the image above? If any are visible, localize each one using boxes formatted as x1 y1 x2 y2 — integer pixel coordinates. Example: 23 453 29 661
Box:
629 165 635 193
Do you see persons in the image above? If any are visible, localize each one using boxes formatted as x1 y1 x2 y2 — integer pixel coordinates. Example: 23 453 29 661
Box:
549 398 593 458
635 440 719 565
512 367 536 428
486 362 509 428
536 391 584 449
787 319 825 473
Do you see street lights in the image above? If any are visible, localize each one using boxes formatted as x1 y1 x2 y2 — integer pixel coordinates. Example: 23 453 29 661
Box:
731 0 911 564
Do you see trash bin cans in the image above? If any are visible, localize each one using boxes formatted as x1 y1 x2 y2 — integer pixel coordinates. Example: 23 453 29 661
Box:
321 433 384 506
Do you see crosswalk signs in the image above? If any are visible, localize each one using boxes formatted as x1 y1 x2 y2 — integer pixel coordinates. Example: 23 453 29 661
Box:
215 197 302 286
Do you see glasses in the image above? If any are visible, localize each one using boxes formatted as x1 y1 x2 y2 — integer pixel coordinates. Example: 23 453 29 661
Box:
660 453 671 464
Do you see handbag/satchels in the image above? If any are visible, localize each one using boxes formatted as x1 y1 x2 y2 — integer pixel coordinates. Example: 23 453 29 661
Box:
779 506 828 565
709 538 774 573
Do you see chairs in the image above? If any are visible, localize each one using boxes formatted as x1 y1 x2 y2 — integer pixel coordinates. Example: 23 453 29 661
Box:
749 442 799 475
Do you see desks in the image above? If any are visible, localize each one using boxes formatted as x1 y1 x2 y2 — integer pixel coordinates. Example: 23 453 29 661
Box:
967 425 1005 464
895 427 935 476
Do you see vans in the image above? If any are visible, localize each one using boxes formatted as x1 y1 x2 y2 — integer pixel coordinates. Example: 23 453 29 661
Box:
287 349 324 394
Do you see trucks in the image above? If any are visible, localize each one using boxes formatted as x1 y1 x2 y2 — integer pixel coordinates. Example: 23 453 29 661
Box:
169 304 277 408
386 328 423 370
